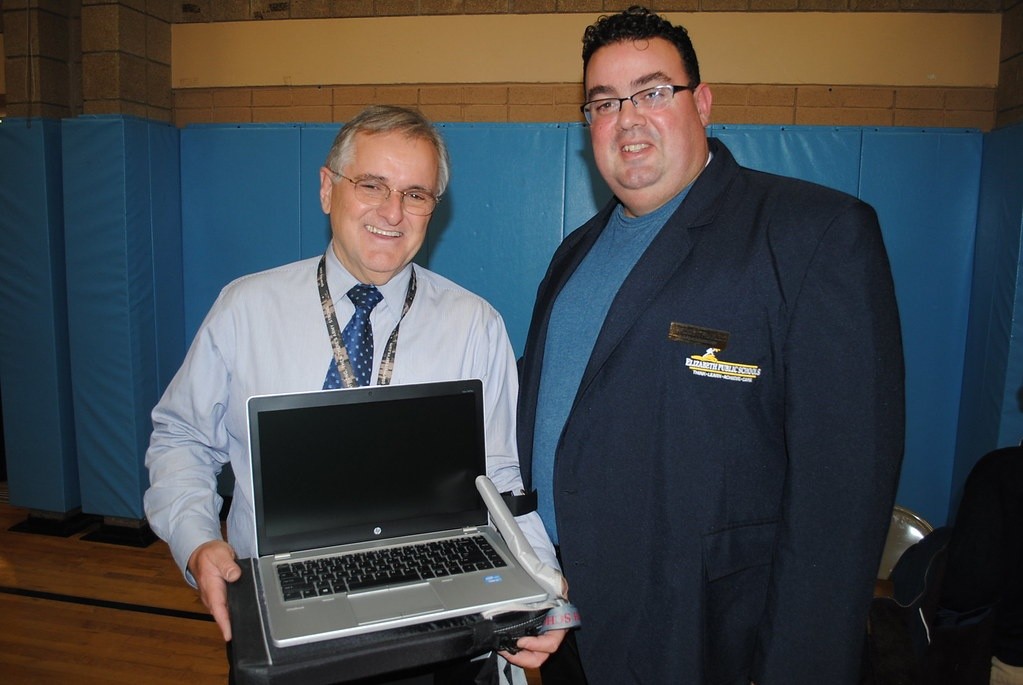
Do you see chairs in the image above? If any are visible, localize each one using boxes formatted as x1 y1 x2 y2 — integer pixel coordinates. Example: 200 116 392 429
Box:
876 505 934 597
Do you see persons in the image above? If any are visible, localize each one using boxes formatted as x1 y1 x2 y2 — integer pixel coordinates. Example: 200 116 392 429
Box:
516 7 906 685
142 109 573 685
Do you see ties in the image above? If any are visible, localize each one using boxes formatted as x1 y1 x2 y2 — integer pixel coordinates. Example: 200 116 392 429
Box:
322 284 384 390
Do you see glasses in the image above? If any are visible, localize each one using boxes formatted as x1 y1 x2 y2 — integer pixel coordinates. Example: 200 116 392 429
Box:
328 169 441 216
580 85 694 126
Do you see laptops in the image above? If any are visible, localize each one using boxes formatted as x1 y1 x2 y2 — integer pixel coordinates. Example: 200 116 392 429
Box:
243 379 548 647
